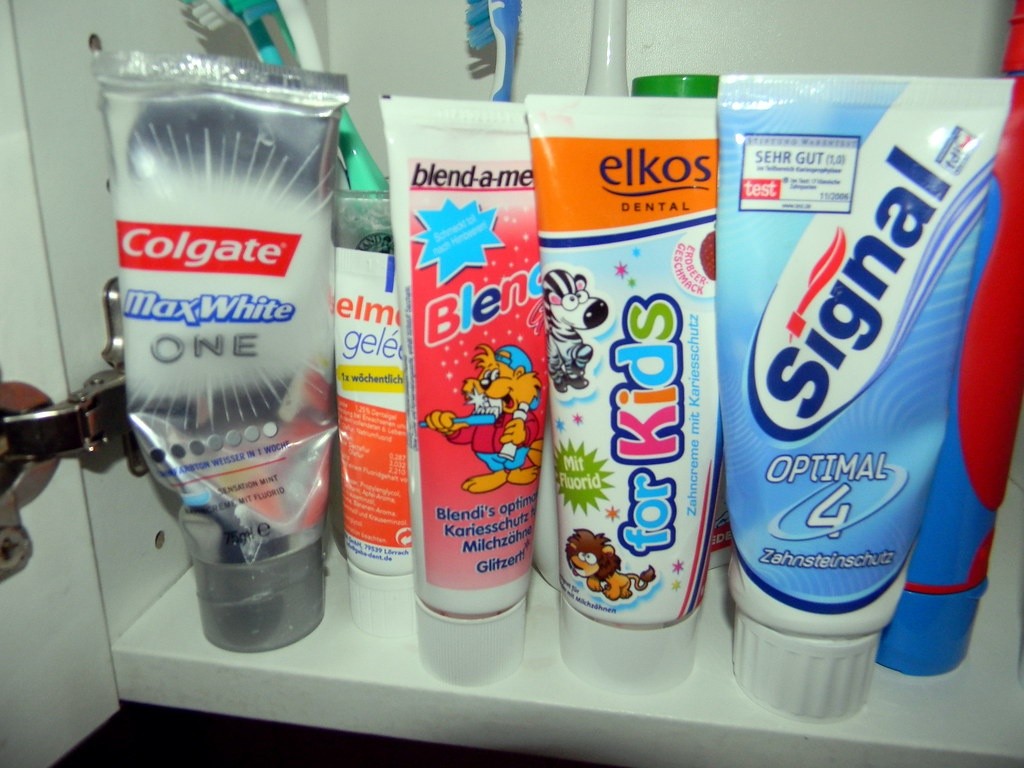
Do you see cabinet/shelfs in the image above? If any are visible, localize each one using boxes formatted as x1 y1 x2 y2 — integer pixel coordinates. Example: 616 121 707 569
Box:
4 0 1023 766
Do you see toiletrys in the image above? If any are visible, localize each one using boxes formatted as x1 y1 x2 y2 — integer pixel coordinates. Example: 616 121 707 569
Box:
94 49 349 654
712 72 1017 726
329 245 423 577
522 89 721 695
377 93 553 688
874 80 1023 679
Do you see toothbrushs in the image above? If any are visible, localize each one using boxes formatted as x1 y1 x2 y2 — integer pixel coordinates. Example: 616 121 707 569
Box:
184 0 346 187
223 0 384 195
463 0 522 106
